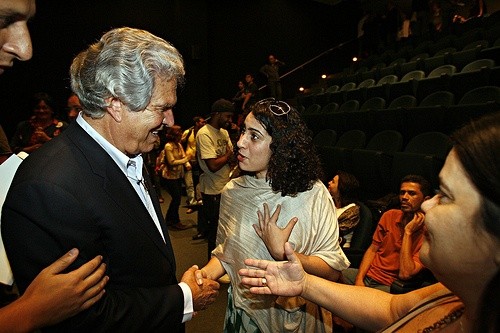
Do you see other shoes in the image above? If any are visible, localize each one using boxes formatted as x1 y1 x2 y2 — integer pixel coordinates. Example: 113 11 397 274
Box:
192 233 203 240
159 198 164 202
168 222 191 230
186 208 194 214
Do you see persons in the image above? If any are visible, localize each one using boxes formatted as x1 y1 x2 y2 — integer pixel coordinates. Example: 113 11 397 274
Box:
0 0 109 333
0 77 84 156
326 171 373 268
194 95 351 333
355 0 500 60
260 54 285 97
0 27 220 333
334 172 430 292
239 111 500 333
143 73 260 260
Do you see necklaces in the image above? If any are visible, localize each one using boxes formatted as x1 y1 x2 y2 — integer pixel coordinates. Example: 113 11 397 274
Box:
421 306 465 333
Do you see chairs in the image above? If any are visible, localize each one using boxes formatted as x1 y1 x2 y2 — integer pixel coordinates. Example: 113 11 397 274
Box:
294 13 500 194
352 201 373 267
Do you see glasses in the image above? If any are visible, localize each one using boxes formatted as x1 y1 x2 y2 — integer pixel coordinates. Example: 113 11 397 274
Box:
259 98 291 121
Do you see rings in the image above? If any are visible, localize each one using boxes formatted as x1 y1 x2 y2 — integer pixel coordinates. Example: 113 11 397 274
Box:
261 277 267 286
